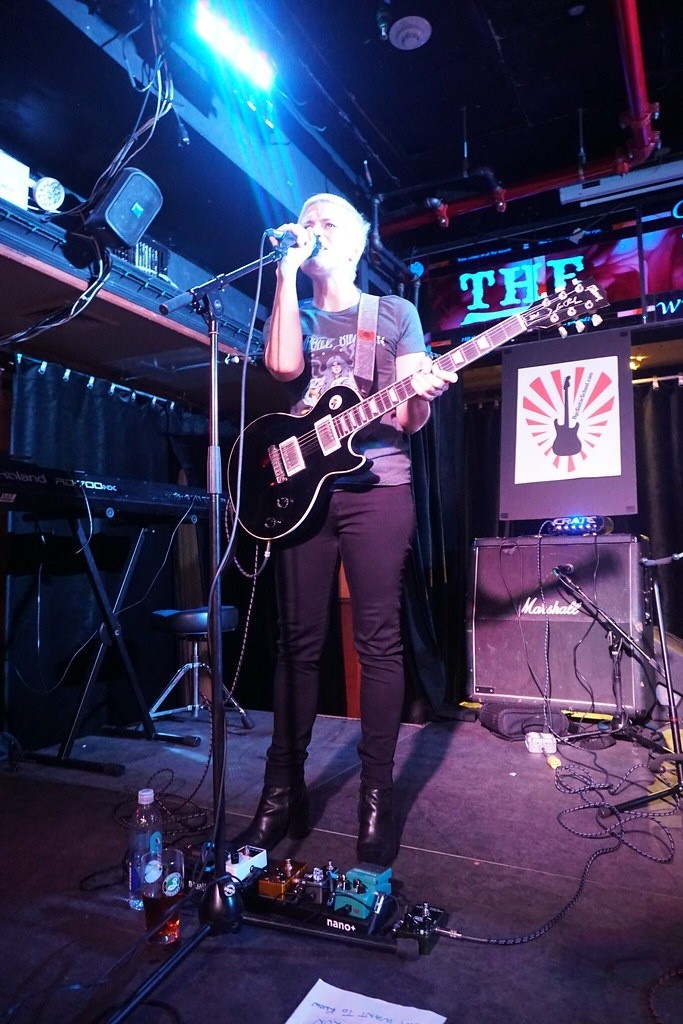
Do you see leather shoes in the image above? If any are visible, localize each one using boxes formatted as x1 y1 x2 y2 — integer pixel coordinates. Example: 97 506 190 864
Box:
356 785 395 868
234 781 308 856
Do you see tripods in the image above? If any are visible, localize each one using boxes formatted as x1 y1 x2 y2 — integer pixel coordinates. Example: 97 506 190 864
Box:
103 244 417 1024
555 576 675 758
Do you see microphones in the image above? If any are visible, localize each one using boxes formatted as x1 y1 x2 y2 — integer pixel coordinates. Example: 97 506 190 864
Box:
551 564 574 574
264 228 321 259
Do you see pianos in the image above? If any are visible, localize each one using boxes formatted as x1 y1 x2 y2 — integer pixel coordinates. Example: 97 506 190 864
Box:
0 456 230 521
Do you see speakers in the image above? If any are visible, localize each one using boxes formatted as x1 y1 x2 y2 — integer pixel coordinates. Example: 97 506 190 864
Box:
86 166 164 250
464 534 657 717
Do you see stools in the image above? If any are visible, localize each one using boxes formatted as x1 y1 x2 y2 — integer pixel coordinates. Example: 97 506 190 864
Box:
138 604 254 733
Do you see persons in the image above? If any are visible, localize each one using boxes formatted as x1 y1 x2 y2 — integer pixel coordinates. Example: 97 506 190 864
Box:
224 191 460 866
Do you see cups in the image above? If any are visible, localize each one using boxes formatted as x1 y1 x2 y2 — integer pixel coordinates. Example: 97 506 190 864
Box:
141 848 185 945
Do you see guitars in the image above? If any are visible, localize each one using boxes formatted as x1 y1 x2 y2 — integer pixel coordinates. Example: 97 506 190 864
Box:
224 274 612 551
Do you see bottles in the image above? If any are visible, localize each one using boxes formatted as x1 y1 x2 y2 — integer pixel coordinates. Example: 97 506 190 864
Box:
129 788 167 912
